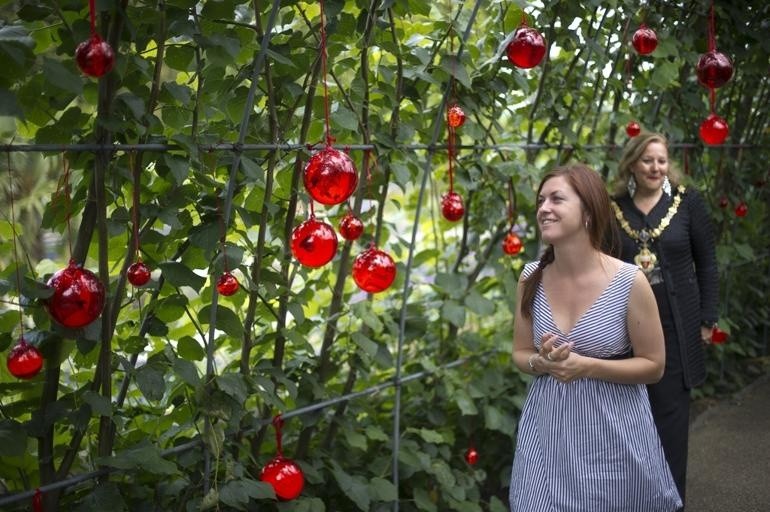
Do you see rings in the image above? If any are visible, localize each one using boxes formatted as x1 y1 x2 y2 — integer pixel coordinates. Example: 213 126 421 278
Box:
547 352 556 362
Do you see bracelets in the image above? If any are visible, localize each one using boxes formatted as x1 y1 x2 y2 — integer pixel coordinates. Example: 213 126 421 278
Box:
528 354 538 373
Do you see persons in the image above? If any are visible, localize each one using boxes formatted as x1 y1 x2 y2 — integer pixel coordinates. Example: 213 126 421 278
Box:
508 165 684 512
605 132 720 512
